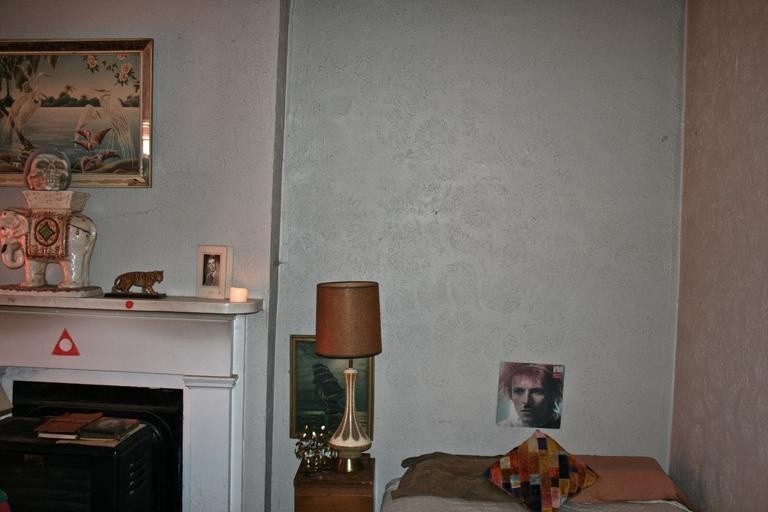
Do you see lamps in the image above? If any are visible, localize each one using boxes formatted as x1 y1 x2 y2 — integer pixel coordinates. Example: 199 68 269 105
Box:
315 280 382 472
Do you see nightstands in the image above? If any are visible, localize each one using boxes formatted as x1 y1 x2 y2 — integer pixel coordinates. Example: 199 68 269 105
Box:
293 458 376 512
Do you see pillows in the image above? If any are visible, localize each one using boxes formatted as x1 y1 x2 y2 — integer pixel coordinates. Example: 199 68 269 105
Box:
482 430 601 512
567 454 677 502
391 451 518 503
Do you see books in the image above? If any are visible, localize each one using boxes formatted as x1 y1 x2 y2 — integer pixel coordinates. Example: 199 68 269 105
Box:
34 412 146 448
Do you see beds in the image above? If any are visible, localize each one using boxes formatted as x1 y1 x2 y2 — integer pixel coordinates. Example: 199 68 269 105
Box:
380 477 693 512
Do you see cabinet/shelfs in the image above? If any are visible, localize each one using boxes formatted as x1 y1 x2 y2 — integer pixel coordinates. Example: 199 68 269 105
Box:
0 381 186 512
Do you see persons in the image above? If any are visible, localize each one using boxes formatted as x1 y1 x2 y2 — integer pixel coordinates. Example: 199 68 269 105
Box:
498 362 561 428
204 256 218 286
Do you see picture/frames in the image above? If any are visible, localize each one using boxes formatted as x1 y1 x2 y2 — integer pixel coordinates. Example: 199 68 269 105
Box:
0 37 154 189
289 334 375 445
194 244 233 300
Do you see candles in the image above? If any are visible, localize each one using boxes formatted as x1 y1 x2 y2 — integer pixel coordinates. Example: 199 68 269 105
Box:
228 287 248 303
